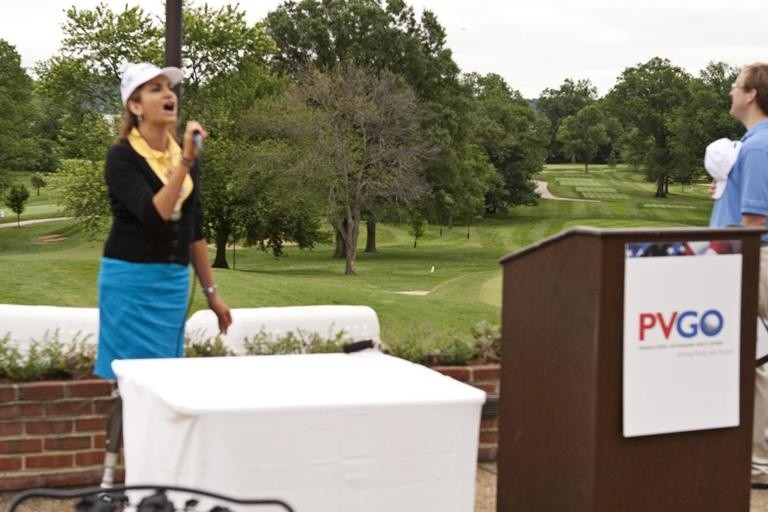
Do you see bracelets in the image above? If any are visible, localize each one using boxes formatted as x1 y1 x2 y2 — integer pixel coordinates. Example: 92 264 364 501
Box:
200 283 220 296
178 151 194 169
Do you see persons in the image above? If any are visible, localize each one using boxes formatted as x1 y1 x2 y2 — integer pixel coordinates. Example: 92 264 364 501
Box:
91 60 232 382
704 66 767 490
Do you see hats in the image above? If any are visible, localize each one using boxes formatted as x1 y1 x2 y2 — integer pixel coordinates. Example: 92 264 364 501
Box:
704 138 742 200
118 62 185 107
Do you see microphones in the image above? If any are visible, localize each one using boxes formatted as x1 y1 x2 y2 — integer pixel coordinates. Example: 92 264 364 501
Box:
184 120 204 151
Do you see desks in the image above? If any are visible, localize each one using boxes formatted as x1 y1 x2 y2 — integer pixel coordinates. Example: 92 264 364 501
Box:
113 350 490 511
